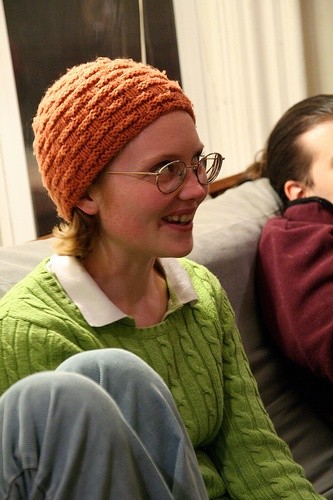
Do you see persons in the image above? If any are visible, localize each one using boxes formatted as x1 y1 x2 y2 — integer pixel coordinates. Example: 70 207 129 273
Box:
0 58 328 500
0 348 210 500
255 95 333 424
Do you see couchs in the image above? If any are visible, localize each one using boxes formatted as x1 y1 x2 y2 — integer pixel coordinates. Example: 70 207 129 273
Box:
0 177 333 500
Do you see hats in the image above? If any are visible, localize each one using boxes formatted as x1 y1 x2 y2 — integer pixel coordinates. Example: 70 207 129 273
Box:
32 57 196 225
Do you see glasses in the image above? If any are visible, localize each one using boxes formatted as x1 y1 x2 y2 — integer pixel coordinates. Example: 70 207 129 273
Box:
99 152 225 195
